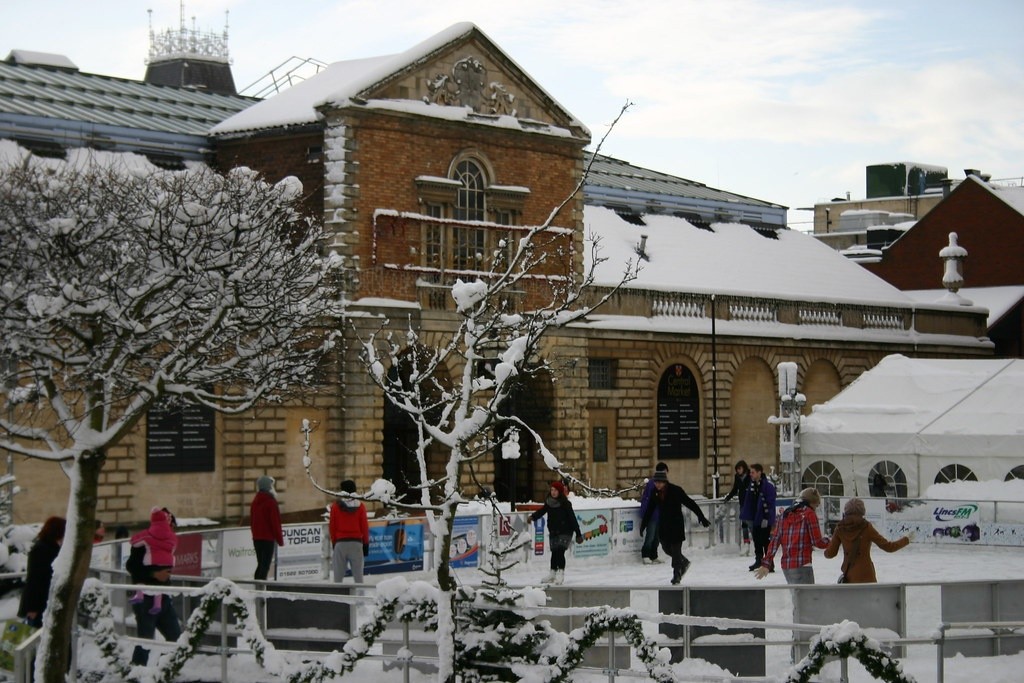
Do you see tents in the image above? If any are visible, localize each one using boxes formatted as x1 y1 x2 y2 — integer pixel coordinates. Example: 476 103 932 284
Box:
787 359 1024 498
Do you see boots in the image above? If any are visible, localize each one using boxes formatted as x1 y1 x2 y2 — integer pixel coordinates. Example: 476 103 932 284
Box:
541 570 555 583
554 570 564 585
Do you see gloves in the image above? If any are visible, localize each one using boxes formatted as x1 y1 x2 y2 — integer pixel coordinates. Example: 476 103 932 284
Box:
363 544 369 556
761 519 768 529
720 497 731 503
577 537 583 544
527 516 534 523
698 516 711 527
640 526 645 536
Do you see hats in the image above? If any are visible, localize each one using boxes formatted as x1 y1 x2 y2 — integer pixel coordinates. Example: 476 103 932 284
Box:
162 508 176 524
258 476 273 491
845 498 865 516
655 471 667 482
151 506 168 522
795 487 820 502
341 480 356 493
551 482 564 493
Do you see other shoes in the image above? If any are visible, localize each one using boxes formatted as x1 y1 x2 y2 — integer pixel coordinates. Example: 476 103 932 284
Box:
150 607 161 614
671 566 686 584
128 594 144 604
653 559 665 563
749 560 760 569
681 556 690 575
642 557 652 565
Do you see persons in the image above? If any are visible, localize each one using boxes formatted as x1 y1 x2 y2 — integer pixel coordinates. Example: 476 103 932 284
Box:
93 520 129 543
639 462 669 565
754 488 829 605
527 482 583 585
17 516 72 676
250 476 284 590
328 480 369 583
125 507 181 683
640 471 711 585
720 460 750 556
823 498 916 584
871 473 894 510
739 464 777 573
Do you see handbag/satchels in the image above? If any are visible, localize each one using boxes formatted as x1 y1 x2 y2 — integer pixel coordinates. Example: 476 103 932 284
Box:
837 574 846 583
0 617 39 672
